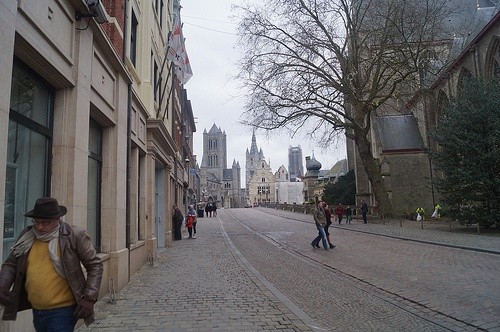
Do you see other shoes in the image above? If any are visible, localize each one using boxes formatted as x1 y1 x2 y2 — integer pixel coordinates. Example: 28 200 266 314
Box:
309 242 336 251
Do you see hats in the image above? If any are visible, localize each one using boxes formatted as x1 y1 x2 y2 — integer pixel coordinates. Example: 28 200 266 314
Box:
24 197 68 219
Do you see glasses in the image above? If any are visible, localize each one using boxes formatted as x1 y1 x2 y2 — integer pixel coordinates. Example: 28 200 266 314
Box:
32 218 51 226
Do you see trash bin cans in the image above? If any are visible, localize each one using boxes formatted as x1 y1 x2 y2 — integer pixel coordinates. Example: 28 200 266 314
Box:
197 209 204 218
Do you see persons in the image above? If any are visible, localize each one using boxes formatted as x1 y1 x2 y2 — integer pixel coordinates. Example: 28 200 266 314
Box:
0 197 104 332
359 199 368 225
416 207 426 221
205 202 216 218
310 201 353 251
172 205 197 240
434 202 442 222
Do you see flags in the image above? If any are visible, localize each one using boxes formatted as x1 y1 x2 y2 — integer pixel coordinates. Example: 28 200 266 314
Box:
165 12 193 84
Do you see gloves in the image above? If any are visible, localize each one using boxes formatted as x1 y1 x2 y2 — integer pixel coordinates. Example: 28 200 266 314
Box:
72 299 95 319
0 287 12 304
